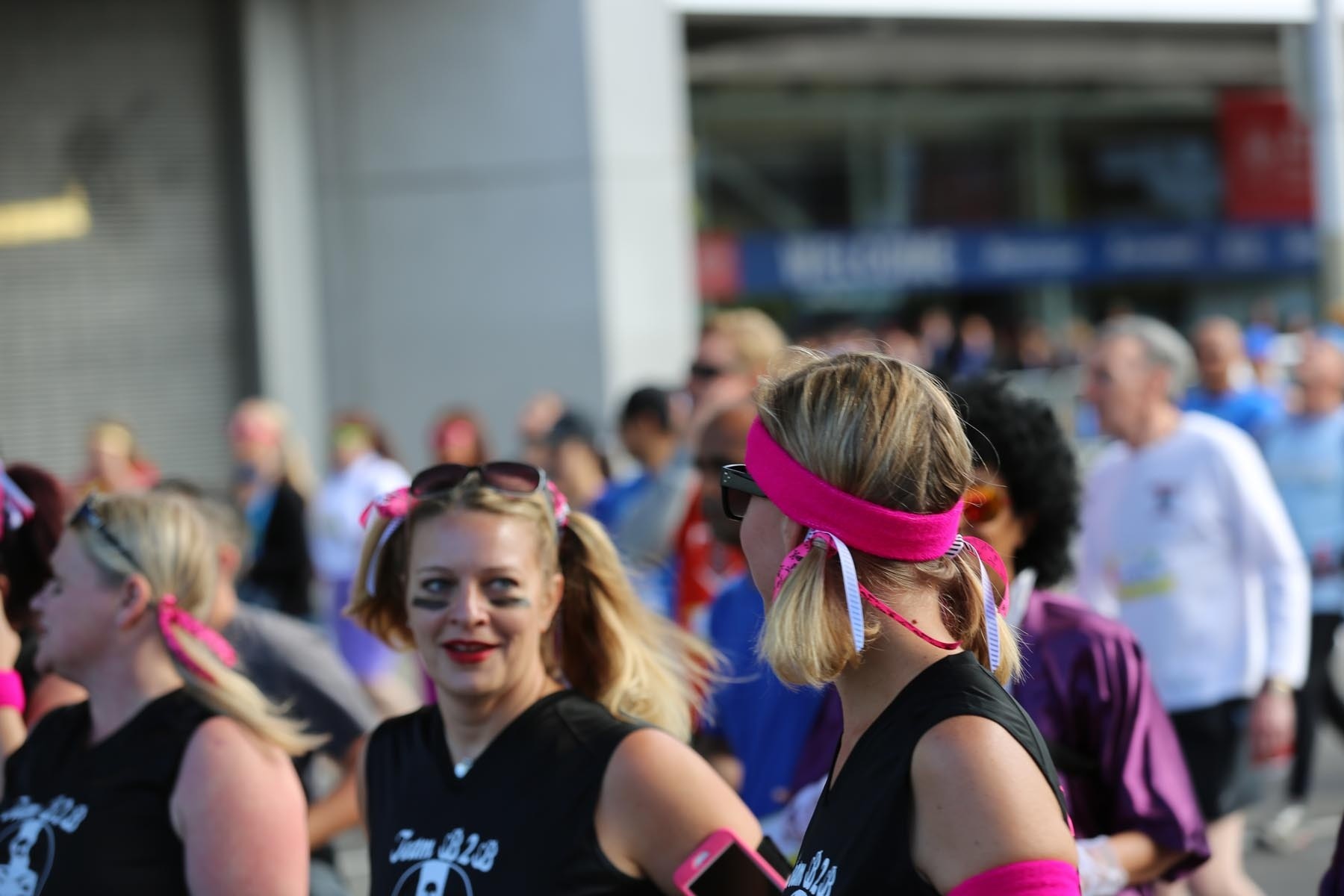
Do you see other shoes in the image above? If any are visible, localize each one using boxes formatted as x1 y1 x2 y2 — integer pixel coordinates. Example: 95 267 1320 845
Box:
1260 802 1313 852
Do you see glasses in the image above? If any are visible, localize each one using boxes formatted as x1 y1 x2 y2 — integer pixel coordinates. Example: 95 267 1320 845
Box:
720 462 766 521
691 364 720 377
403 460 560 561
68 487 159 603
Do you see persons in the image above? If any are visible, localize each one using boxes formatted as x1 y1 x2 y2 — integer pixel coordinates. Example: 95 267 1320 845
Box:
0 309 1344 896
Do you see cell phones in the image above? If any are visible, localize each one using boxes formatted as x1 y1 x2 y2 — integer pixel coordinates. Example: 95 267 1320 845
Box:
674 827 788 896
